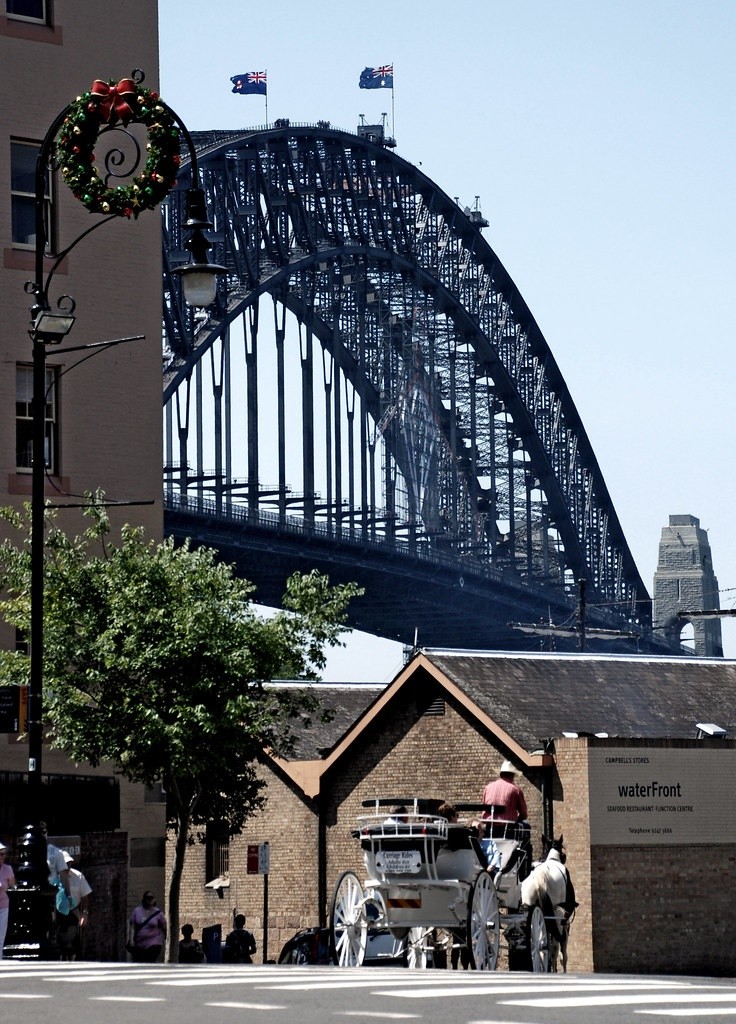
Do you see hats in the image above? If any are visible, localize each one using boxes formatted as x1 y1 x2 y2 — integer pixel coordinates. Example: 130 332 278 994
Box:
0 842 7 851
62 851 74 863
500 758 522 776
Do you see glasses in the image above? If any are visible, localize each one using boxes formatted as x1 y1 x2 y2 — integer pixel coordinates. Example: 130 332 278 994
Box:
145 895 155 900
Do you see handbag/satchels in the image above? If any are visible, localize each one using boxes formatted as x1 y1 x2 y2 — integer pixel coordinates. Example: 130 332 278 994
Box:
124 921 144 953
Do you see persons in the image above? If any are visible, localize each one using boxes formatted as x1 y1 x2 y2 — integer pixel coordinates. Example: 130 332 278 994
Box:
0 843 92 962
178 923 205 963
384 802 501 879
482 760 535 880
225 913 256 964
125 891 167 963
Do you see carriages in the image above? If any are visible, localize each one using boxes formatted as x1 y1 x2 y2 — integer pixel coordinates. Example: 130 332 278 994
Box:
326 796 576 972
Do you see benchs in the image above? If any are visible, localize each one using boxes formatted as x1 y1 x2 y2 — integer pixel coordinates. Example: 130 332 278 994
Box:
351 799 531 894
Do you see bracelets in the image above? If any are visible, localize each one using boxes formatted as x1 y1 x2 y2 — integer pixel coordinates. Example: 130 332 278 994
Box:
84 910 89 915
67 896 72 899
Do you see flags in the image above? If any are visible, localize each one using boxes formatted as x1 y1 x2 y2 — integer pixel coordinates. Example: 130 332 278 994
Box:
358 65 393 89
231 71 267 95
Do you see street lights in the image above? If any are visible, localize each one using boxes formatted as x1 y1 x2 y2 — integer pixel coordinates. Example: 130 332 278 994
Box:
1 69 232 958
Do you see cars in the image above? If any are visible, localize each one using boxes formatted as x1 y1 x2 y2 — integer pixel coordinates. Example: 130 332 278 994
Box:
275 925 330 966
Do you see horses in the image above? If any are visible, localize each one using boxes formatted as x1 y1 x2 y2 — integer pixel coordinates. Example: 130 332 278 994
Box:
521 833 571 973
